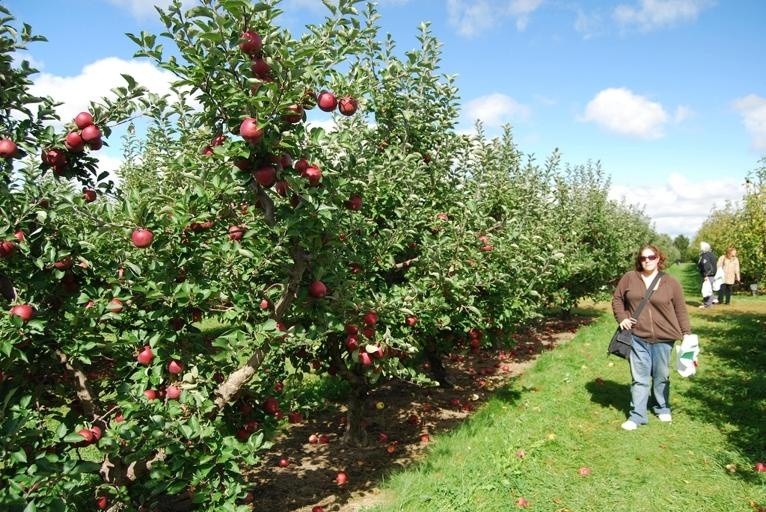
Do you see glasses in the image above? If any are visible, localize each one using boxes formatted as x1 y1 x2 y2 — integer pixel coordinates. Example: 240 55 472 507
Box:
638 256 656 261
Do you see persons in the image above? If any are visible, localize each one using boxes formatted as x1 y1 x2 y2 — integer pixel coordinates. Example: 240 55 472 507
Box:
717 247 740 304
608 243 699 431
698 241 717 308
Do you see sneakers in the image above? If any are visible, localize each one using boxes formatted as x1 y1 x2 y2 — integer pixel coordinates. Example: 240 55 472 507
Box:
658 414 672 422
699 299 729 311
621 420 638 431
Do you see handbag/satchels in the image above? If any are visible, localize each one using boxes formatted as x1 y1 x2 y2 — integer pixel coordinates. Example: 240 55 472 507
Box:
607 326 633 360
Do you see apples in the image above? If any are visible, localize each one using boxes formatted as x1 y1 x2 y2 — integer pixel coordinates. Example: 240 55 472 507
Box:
753 461 766 473
0 31 590 512
